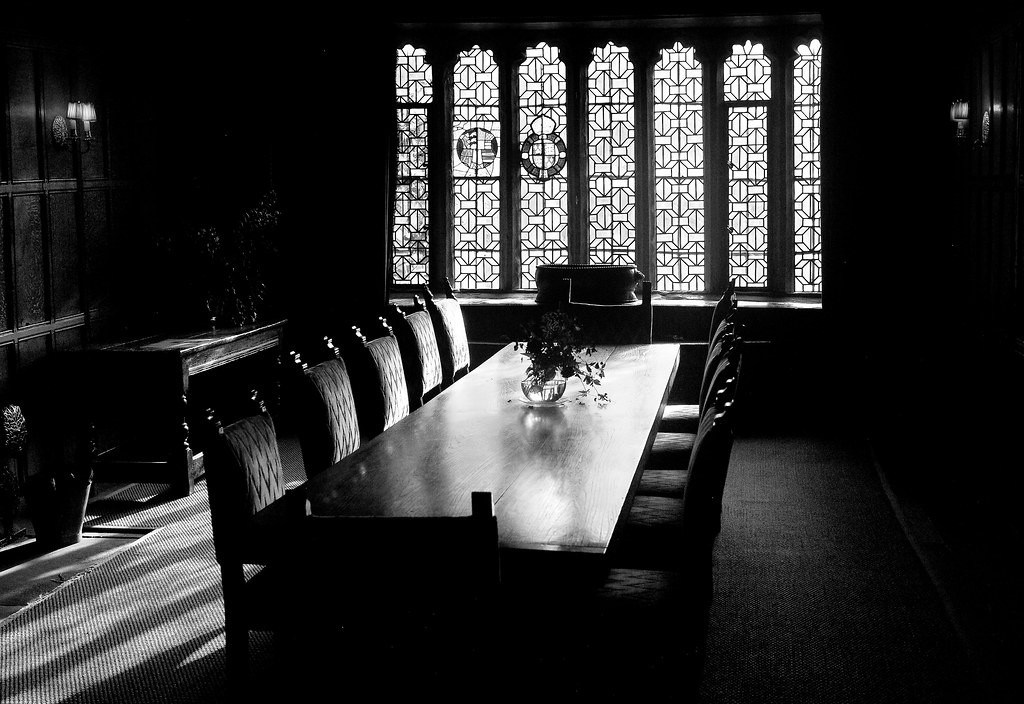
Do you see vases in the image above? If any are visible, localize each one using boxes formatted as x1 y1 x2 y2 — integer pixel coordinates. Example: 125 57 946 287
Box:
521 354 565 400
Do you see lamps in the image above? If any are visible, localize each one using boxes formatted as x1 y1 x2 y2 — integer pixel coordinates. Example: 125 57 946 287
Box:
53 100 97 143
947 99 983 159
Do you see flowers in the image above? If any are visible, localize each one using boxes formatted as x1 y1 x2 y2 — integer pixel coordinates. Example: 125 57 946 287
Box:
514 321 611 405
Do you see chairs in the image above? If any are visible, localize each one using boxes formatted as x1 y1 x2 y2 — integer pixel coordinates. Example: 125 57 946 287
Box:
196 276 495 703
585 298 760 703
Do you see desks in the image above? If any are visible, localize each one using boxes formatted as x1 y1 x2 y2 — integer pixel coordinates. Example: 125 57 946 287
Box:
65 313 287 488
252 340 682 702
